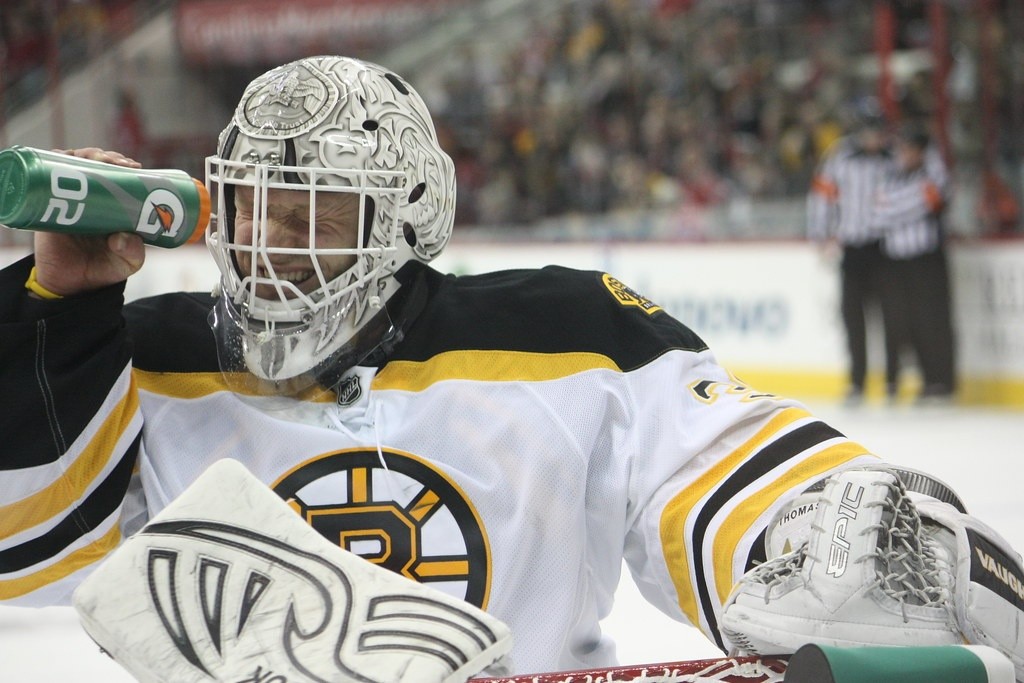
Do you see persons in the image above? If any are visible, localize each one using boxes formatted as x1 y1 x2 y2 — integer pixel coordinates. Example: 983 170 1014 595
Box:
429 1 1024 409
1 55 1024 683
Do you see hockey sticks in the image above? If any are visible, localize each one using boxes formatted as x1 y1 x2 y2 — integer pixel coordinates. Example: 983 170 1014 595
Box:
468 654 789 683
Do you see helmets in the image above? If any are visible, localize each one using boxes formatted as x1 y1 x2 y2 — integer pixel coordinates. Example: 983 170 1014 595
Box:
842 95 892 132
891 120 934 140
202 55 458 412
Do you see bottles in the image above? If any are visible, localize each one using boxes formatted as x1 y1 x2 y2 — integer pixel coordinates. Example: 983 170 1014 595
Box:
0 146 212 248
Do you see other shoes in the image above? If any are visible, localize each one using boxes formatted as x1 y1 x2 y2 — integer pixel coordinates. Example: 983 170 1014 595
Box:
847 386 862 409
914 394 953 411
884 390 898 406
